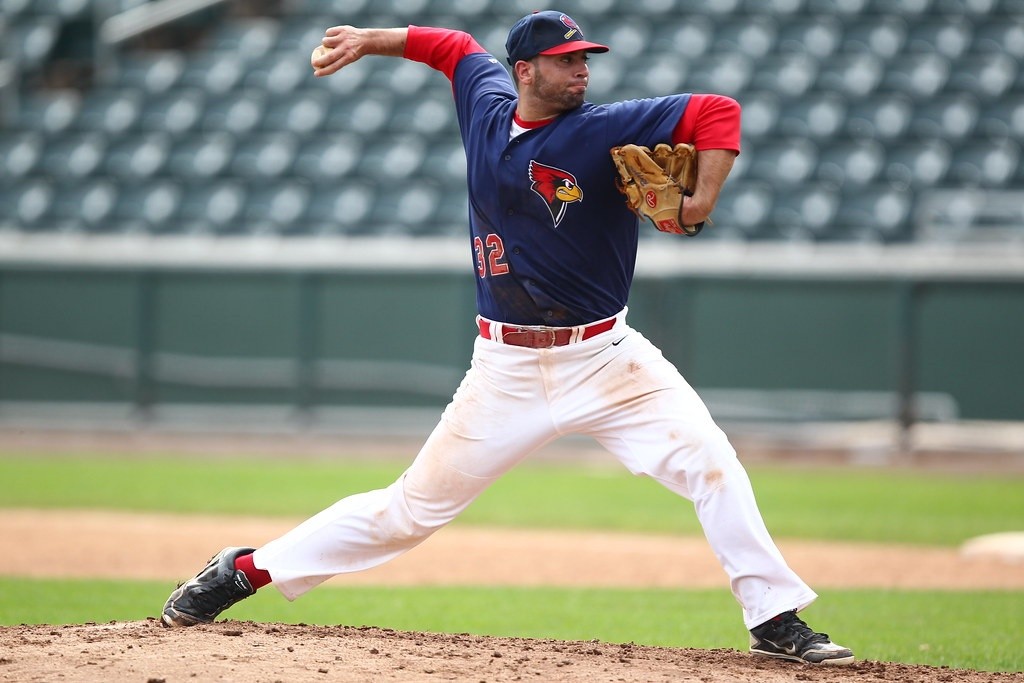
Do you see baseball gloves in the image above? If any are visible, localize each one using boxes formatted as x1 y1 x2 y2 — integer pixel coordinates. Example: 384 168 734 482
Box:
611 143 704 237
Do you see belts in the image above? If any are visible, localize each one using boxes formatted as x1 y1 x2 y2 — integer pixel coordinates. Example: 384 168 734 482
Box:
479 317 616 349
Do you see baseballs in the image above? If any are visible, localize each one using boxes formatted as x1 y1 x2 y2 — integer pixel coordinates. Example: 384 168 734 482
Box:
311 46 335 71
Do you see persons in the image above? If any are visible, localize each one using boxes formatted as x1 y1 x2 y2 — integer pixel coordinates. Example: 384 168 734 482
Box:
162 10 855 667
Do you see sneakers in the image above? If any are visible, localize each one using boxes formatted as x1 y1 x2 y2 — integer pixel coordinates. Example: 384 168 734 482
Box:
161 547 256 629
750 608 854 667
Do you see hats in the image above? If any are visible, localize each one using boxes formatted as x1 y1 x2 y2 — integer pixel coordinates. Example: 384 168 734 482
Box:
505 10 609 66
